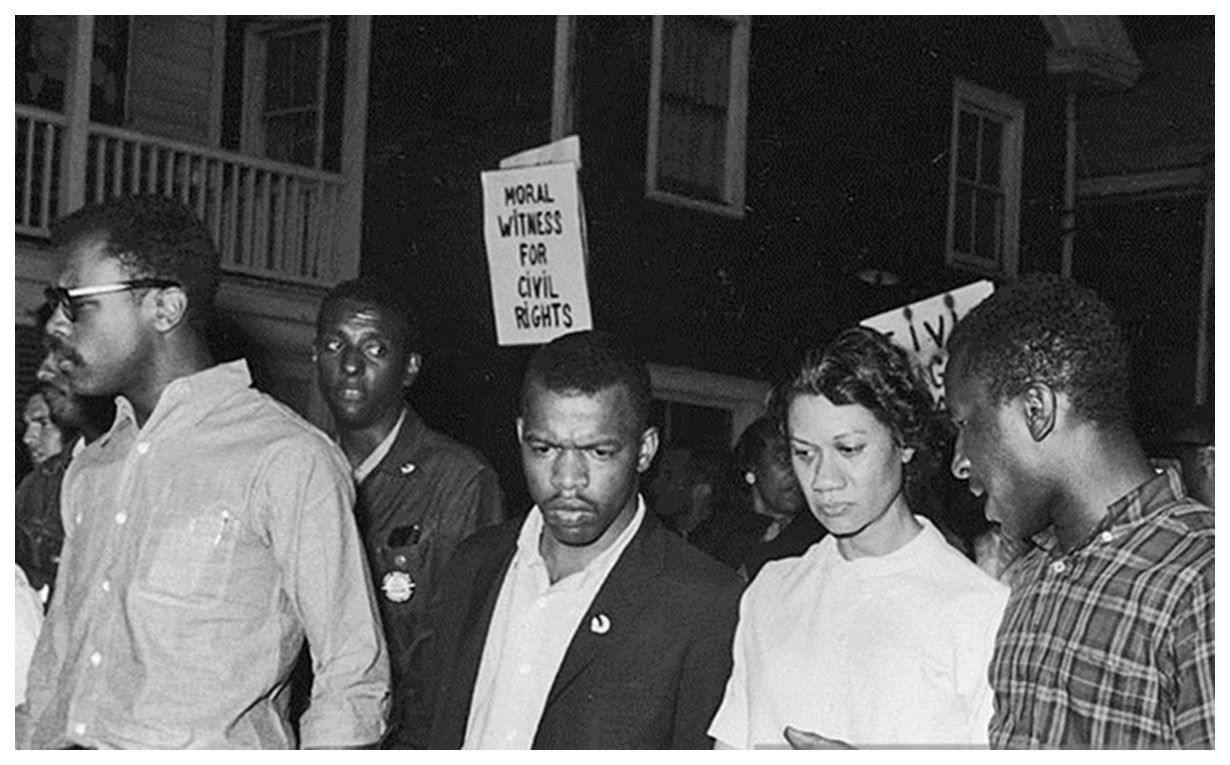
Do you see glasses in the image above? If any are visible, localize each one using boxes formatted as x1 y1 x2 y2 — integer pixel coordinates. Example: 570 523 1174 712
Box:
40 278 182 323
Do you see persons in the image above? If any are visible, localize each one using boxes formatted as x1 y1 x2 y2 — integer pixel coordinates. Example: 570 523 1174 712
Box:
13 194 1216 751
